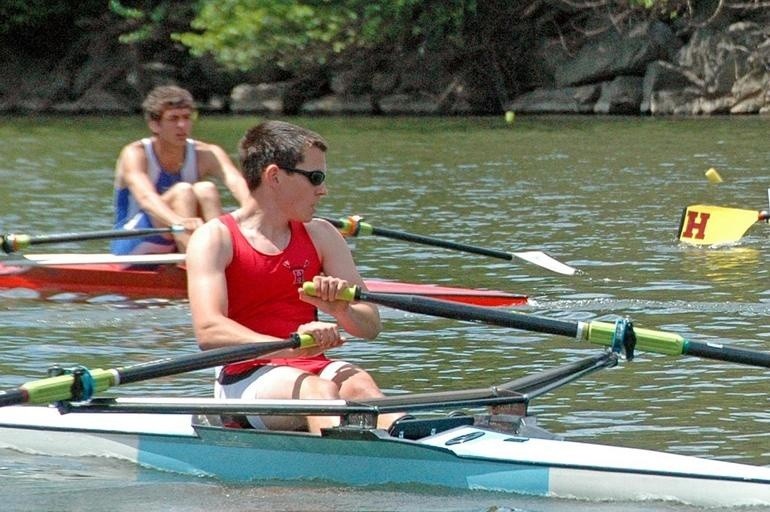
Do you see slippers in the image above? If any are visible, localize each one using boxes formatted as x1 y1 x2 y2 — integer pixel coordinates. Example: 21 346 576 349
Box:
384 410 467 437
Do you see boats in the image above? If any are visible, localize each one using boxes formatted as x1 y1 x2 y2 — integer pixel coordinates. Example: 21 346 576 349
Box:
2 260 527 306
0 390 769 508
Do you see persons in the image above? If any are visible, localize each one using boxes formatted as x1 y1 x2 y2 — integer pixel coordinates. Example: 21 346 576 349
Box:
185 119 468 435
112 85 252 255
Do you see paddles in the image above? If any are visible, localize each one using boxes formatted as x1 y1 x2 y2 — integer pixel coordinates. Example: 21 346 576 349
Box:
313 215 588 277
678 203 769 246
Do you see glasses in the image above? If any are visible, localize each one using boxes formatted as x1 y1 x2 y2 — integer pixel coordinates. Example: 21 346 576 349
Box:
275 165 327 186
